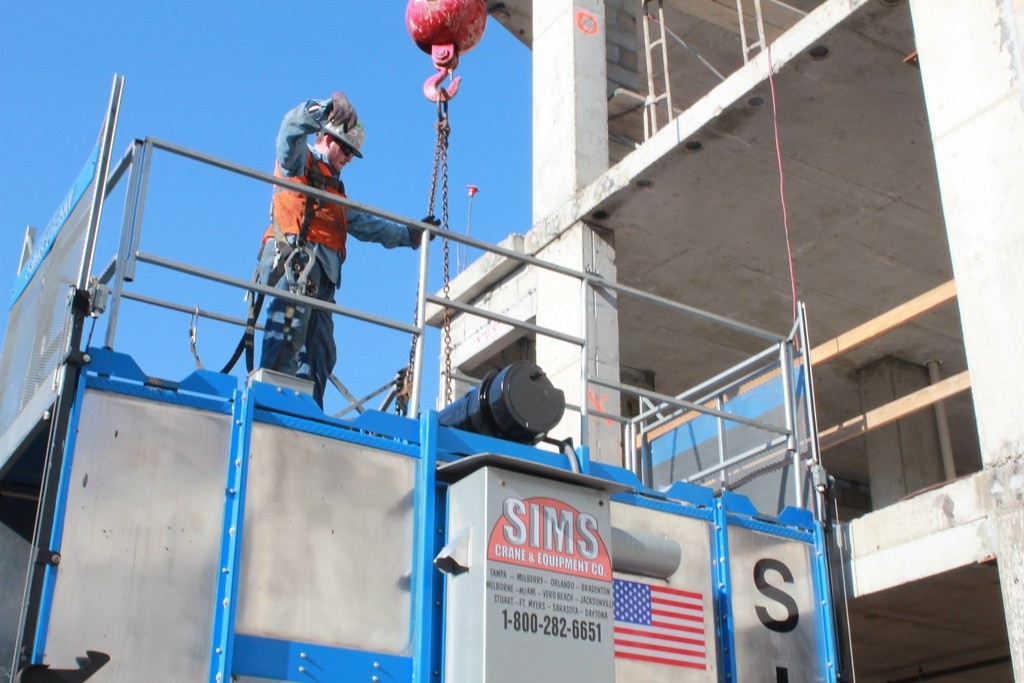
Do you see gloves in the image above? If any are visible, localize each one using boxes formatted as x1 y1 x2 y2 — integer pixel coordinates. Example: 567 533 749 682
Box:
408 215 441 250
330 91 357 133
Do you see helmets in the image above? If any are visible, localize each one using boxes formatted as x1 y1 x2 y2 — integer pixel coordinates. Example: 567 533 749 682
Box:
324 112 364 160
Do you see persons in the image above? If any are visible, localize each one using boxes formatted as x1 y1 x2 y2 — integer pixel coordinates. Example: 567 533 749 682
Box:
260 90 441 414
